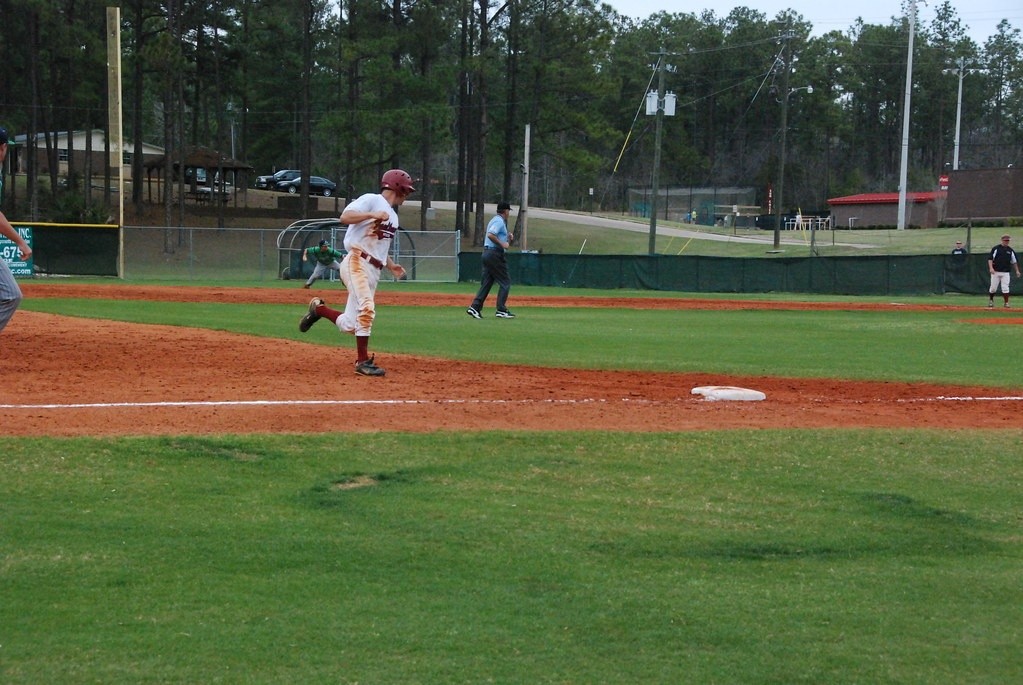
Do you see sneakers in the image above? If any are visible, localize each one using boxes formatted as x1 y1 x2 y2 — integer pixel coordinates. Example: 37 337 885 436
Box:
466 304 483 319
354 353 385 378
495 307 515 319
299 297 324 332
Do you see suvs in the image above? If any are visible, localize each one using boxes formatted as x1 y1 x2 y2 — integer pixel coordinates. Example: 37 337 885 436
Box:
255 169 301 191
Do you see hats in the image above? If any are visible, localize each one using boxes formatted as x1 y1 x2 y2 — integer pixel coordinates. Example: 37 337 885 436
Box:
497 203 513 211
320 241 329 247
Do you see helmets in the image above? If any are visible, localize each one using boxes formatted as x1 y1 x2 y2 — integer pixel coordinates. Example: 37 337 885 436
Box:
381 169 416 197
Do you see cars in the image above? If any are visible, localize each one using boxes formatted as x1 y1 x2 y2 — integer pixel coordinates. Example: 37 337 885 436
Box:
184 167 219 186
276 175 337 197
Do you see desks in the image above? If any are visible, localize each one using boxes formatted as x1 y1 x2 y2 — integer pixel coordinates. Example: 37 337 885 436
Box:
196 186 231 208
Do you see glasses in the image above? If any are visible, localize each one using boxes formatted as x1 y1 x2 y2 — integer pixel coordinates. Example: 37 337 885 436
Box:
1002 239 1009 242
957 243 962 244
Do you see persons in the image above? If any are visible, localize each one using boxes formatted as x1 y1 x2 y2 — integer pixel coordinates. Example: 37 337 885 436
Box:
299 170 416 376
796 212 802 230
988 234 1020 308
465 202 516 319
724 216 727 227
952 241 967 254
0 127 30 330
303 240 347 288
687 208 698 224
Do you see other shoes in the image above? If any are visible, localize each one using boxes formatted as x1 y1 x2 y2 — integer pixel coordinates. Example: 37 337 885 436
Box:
1004 303 1010 308
304 285 310 289
989 300 994 307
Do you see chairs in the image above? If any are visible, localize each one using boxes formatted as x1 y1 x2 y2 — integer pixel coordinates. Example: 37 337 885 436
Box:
195 192 209 208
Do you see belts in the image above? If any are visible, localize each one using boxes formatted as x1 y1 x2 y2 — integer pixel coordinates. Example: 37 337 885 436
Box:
483 246 504 251
360 252 383 270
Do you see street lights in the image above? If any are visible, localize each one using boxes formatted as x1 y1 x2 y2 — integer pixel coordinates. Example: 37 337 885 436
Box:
773 86 814 251
942 55 976 171
